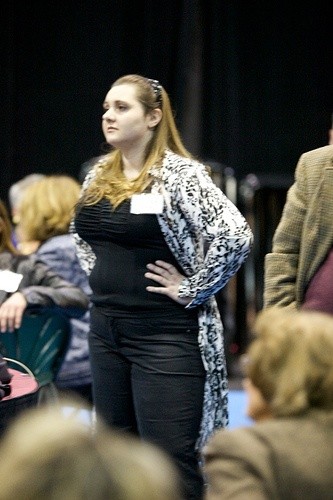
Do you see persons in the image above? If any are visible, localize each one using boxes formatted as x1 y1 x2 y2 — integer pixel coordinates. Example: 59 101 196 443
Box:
263 145 333 316
70 75 254 500
202 305 333 500
10 174 92 407
0 200 89 333
